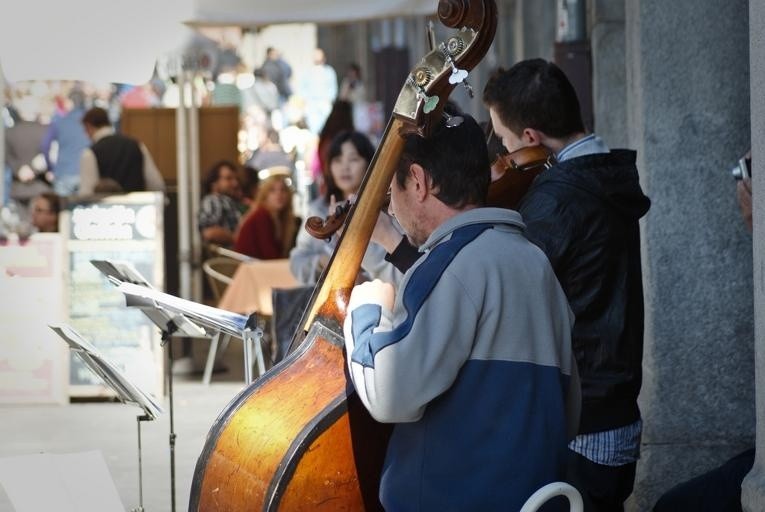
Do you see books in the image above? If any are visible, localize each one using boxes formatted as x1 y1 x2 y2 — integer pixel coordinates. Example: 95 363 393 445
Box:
117 279 249 340
48 322 166 421
113 261 209 339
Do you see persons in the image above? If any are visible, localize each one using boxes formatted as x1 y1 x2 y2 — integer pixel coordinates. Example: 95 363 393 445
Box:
0 43 405 297
338 106 589 511
478 57 654 512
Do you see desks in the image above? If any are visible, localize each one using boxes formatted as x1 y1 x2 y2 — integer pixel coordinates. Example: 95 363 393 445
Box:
218 257 305 322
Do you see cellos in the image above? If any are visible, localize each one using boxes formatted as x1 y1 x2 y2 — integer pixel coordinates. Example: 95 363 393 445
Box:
188 0 497 510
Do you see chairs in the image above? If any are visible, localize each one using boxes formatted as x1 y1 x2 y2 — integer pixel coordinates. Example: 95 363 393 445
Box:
202 255 250 392
204 241 258 263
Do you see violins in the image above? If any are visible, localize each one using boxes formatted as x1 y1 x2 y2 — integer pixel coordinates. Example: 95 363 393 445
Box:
490 146 557 207
